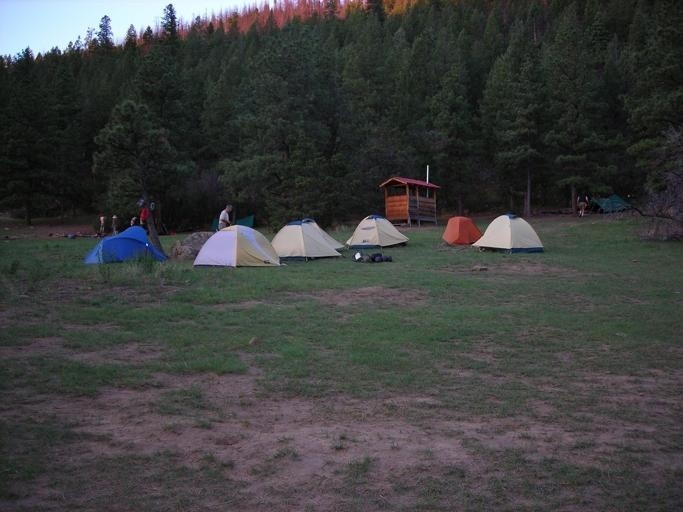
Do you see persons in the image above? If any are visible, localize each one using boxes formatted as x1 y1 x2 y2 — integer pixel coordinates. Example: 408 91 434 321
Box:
577 191 589 217
99 215 146 235
218 203 233 230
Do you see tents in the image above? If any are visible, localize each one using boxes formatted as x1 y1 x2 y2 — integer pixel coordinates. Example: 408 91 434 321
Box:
192 225 280 268
302 218 345 251
471 212 544 254
271 220 342 262
345 215 410 249
442 216 482 245
83 226 166 264
589 194 632 214
